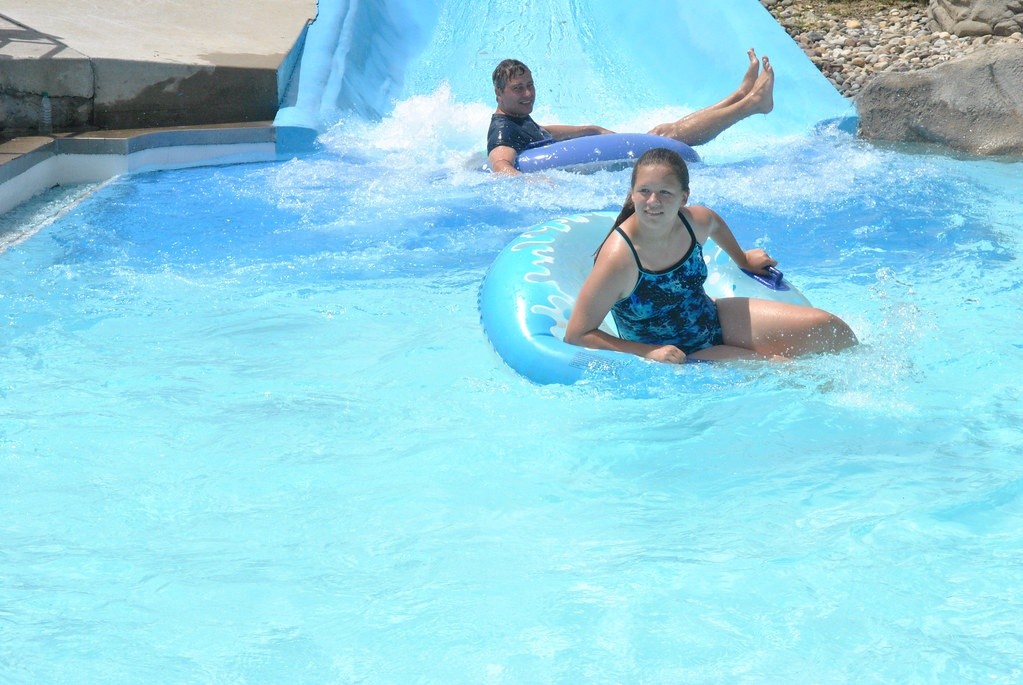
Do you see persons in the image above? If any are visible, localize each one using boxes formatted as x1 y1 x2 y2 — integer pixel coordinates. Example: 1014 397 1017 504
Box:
566 148 859 364
486 48 774 178
